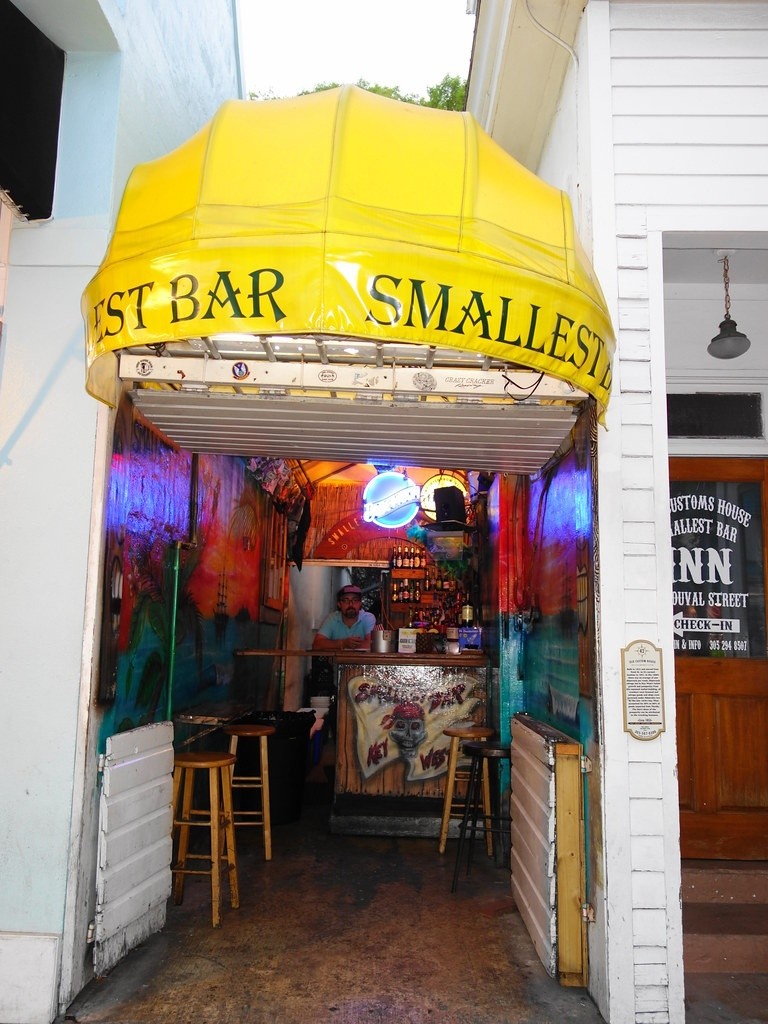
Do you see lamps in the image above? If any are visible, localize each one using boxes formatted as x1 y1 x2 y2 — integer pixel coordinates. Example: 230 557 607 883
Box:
707 249 752 359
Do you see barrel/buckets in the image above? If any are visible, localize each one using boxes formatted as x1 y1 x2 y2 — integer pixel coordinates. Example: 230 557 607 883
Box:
311 696 332 745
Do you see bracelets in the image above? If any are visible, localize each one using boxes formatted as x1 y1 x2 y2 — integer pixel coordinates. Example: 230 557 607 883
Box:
338 640 342 649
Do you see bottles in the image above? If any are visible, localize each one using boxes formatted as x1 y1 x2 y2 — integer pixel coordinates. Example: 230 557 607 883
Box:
390 545 475 642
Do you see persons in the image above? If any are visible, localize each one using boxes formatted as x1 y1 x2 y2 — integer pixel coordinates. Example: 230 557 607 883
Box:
315 586 377 743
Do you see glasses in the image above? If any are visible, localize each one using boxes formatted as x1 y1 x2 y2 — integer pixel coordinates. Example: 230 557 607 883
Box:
339 597 361 605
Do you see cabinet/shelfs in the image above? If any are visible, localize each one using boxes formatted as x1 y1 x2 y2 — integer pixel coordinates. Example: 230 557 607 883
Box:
391 569 449 613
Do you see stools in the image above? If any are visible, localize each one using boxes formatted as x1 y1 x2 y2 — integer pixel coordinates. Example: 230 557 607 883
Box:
221 724 275 860
439 727 494 856
452 741 511 892
172 751 241 929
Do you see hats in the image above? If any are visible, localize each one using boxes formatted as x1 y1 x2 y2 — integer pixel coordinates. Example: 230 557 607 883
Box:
337 585 363 598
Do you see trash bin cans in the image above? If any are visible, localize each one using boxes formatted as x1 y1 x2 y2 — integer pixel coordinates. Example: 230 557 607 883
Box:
228 710 316 828
